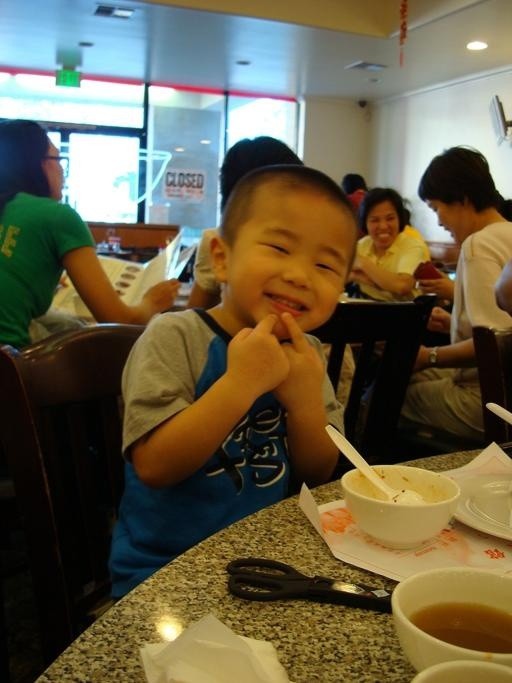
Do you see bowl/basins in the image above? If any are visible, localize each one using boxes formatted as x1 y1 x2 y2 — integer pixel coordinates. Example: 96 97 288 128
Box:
342 461 462 549
391 564 512 671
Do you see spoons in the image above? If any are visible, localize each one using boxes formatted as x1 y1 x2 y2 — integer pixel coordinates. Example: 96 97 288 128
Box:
322 421 427 508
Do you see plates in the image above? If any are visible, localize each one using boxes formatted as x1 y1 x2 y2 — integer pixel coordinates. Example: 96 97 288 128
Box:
451 472 511 545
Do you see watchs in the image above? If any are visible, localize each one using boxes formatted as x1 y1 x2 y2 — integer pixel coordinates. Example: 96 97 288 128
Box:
428 345 438 365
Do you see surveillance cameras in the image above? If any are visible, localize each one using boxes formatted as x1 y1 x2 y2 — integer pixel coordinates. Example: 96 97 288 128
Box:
358 100 367 108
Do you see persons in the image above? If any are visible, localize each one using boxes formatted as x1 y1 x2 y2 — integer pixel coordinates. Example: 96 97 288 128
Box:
385 145 512 446
103 162 363 604
345 186 435 355
340 171 369 209
416 265 454 303
183 133 306 311
1 116 182 356
494 254 511 318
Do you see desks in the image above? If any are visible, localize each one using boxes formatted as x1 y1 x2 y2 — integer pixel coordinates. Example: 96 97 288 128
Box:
83 221 179 262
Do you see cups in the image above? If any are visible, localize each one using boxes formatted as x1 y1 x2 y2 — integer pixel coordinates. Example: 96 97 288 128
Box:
109 238 121 251
347 278 361 296
405 662 512 683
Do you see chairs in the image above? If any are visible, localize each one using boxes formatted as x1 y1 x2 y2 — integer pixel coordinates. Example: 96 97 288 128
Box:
0 323 146 667
469 323 511 443
304 293 437 483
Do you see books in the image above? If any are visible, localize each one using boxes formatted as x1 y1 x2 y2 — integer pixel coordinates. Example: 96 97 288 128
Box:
43 228 197 324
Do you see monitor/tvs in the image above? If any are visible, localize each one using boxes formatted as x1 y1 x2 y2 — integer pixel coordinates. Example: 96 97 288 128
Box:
488 96 508 145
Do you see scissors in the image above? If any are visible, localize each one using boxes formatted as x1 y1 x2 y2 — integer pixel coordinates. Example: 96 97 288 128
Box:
227 558 393 614
